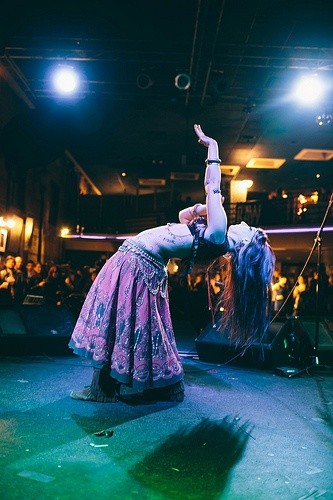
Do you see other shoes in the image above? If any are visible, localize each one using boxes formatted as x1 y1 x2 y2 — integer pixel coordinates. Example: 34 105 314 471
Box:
142 383 185 402
70 388 120 402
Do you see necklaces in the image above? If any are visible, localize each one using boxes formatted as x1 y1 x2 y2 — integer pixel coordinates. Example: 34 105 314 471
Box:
166 218 207 238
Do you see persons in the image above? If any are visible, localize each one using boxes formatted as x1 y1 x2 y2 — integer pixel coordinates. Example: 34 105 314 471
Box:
67 121 276 404
1 251 333 320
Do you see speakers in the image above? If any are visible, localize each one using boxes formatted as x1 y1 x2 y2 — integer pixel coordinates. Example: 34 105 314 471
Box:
195 312 312 371
0 303 78 357
288 316 333 365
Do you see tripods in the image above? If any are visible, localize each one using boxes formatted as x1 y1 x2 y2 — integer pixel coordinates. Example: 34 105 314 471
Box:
269 193 333 379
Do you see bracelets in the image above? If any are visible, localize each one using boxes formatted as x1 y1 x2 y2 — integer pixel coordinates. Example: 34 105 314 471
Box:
203 156 223 166
203 189 224 198
187 204 202 218
